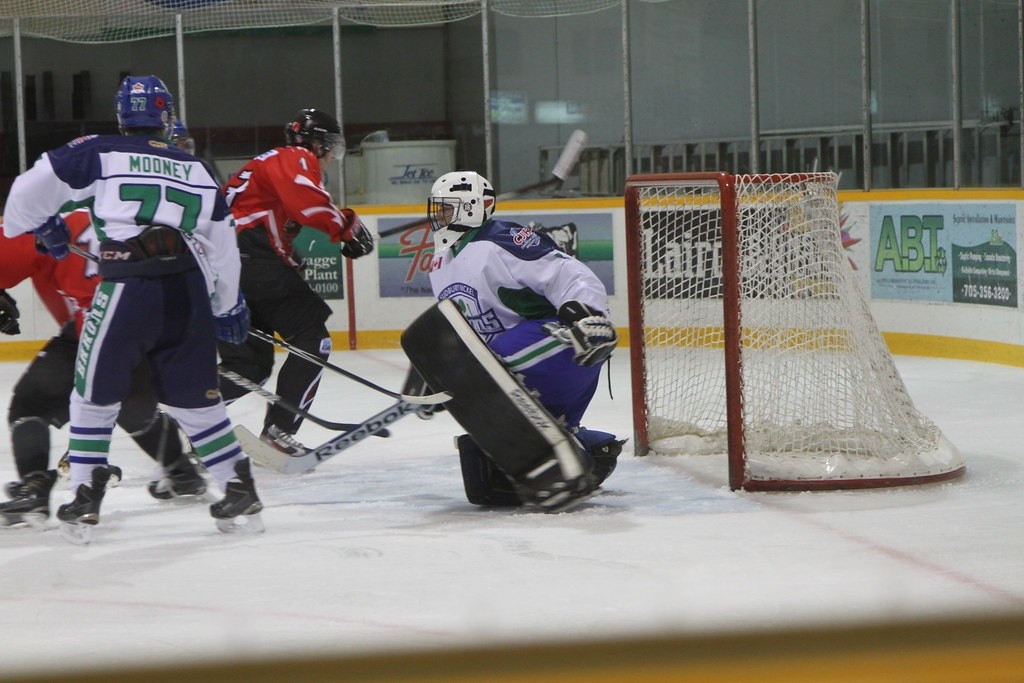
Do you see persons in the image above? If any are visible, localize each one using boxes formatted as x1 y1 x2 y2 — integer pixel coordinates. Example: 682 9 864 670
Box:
402 172 623 505
0 75 267 546
212 109 374 472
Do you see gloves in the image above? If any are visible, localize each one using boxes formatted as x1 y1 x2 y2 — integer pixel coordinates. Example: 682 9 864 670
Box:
212 288 249 345
0 289 22 335
329 207 372 259
553 300 617 366
27 213 72 259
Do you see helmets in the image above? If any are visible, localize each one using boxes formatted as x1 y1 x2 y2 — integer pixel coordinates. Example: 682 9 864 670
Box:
286 107 341 157
166 118 188 139
114 75 172 128
426 171 496 252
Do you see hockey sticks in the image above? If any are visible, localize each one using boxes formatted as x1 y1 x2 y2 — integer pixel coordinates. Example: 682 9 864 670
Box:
218 367 392 438
35 229 453 406
230 400 425 477
371 127 589 242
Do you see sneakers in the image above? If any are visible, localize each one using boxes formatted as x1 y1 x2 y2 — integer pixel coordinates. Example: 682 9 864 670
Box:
253 423 316 475
6 480 22 498
209 455 265 533
148 454 213 503
55 465 124 546
0 469 57 527
